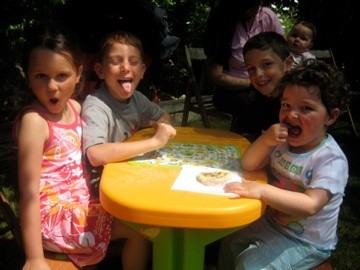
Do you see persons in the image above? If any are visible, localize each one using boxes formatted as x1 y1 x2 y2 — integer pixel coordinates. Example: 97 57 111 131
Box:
216 58 349 269
242 31 294 143
285 16 316 71
81 31 179 197
14 26 150 270
203 0 285 144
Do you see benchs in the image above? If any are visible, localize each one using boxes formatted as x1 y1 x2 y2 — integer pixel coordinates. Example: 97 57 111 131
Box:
182 45 338 130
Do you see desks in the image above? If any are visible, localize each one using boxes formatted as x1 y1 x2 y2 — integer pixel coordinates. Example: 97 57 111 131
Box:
99 127 267 270
